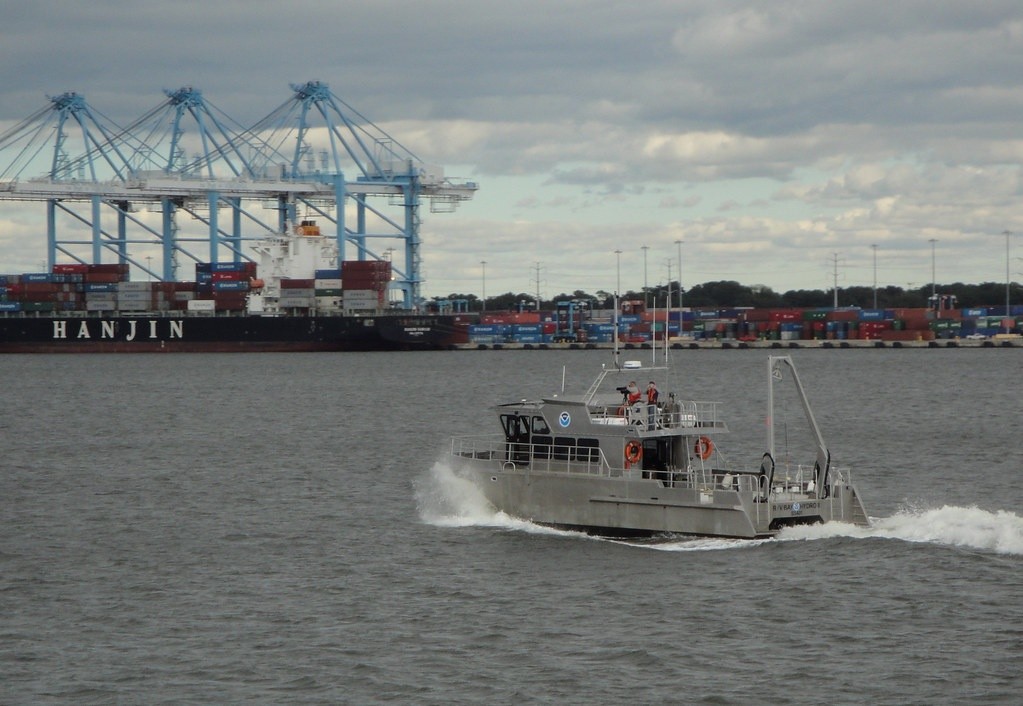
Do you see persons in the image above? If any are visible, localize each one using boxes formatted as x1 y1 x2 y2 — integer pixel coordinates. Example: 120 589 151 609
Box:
642 381 659 430
626 381 641 425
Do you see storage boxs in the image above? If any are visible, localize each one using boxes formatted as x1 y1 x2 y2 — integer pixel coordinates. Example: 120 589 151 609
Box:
1 255 394 316
468 297 1022 339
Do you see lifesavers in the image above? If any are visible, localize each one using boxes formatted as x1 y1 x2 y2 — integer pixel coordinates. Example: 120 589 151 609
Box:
626 441 643 462
695 437 713 458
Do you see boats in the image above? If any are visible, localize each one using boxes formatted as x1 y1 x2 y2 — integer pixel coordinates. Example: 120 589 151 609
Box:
0 299 473 348
445 292 874 541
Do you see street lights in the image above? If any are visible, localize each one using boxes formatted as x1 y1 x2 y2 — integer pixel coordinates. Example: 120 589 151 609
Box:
639 246 651 312
927 238 939 308
868 242 880 311
145 256 154 280
613 249 624 316
674 240 685 336
480 260 488 311
999 230 1017 336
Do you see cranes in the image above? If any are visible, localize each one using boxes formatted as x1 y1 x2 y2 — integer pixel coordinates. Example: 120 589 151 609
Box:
0 74 482 312
